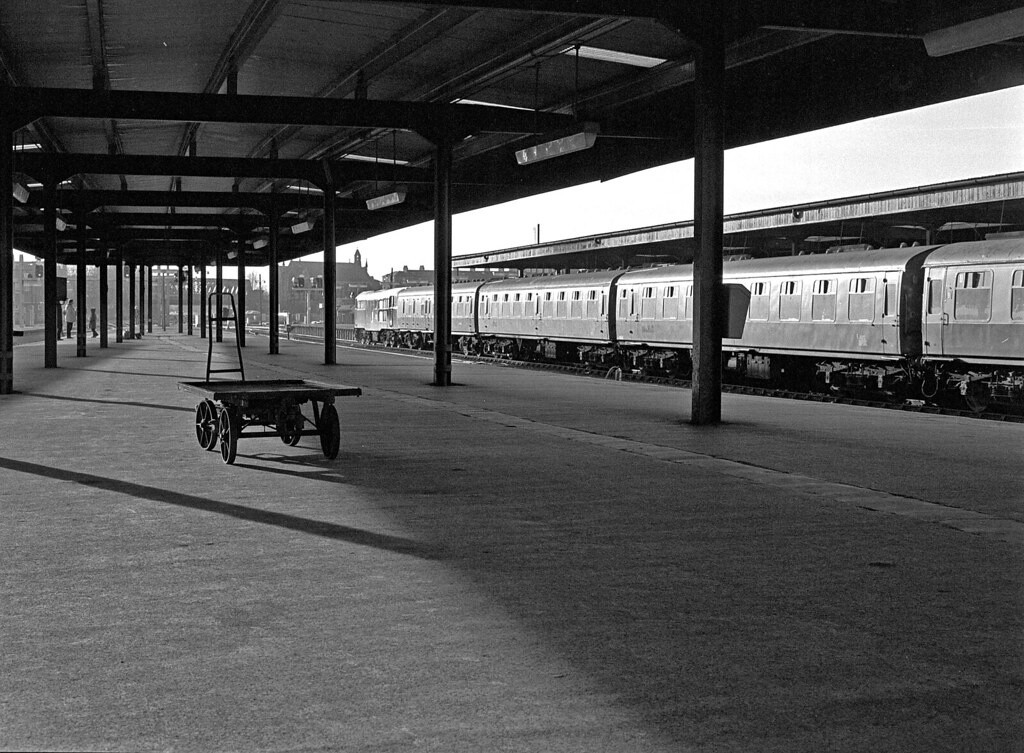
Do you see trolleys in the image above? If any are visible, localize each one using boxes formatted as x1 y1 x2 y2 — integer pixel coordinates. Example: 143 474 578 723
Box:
178 292 362 465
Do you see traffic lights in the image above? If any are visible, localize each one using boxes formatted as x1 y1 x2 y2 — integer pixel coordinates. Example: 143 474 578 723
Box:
37 264 43 280
125 267 129 280
312 278 322 288
299 277 304 287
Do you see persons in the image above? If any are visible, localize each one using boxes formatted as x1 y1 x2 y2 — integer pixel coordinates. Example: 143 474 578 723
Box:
57 301 63 341
65 299 76 338
89 308 99 338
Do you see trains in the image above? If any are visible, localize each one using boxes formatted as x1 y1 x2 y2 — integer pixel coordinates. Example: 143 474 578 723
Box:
354 238 1024 406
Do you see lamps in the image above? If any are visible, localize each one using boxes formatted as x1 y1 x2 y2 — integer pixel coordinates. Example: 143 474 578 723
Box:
515 43 600 165
56 182 66 232
366 130 407 210
253 213 267 249
227 251 238 259
923 6 1024 57
13 131 29 203
291 178 316 234
